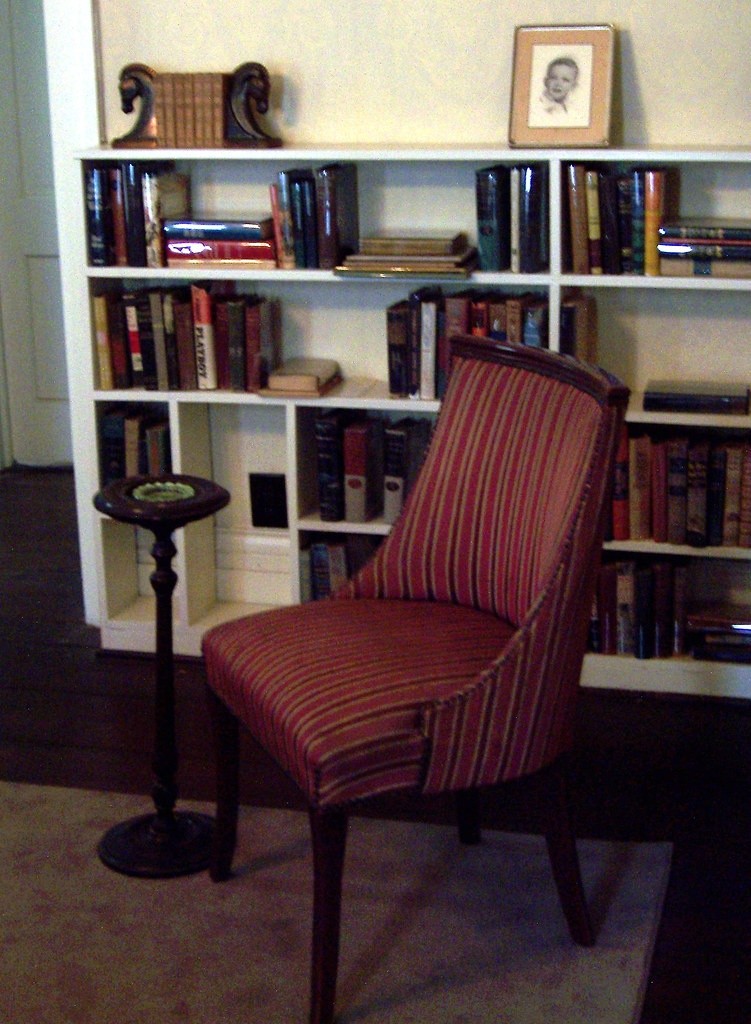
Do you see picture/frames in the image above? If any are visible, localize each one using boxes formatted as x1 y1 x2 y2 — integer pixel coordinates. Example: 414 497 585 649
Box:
508 21 615 148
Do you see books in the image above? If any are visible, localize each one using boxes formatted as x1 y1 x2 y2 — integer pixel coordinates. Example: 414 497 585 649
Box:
161 216 278 271
99 411 171 488
587 554 751 666
572 164 677 276
154 70 237 148
560 295 598 369
606 425 751 548
84 164 190 269
259 357 342 399
387 286 547 401
93 280 281 392
659 217 751 280
476 163 548 271
331 231 477 280
641 378 751 416
267 161 359 270
315 407 434 524
300 540 355 605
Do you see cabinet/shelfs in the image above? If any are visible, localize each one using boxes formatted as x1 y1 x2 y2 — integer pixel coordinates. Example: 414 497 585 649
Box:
71 141 751 700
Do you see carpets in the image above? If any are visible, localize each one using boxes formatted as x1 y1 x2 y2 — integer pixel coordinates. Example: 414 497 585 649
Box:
0 780 674 1024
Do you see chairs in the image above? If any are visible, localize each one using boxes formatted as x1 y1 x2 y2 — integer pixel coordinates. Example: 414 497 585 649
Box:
200 337 633 1024
